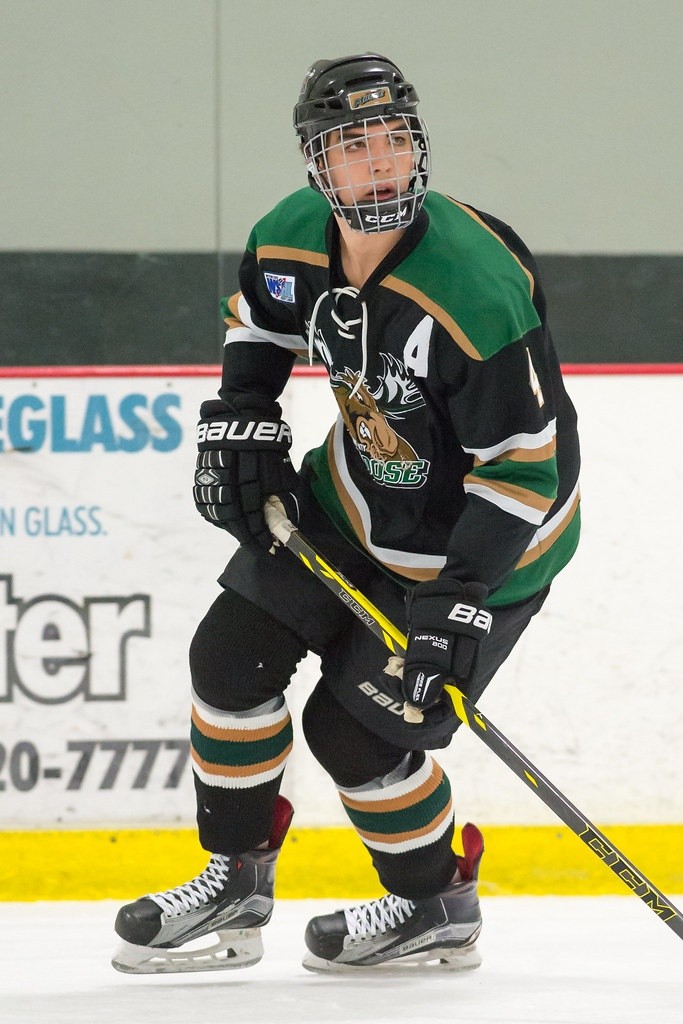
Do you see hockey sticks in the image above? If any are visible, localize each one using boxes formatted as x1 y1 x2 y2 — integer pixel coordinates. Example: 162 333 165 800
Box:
261 498 683 936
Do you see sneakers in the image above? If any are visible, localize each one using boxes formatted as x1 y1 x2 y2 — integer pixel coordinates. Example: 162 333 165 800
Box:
299 824 488 975
110 795 293 974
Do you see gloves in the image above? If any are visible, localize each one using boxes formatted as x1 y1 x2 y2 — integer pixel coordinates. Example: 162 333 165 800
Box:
336 574 493 748
194 397 303 551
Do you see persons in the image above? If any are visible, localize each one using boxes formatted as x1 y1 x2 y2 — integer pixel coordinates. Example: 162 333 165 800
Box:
109 52 580 975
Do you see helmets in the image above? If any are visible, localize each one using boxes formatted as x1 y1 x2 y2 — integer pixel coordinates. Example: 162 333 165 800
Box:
296 53 432 229
